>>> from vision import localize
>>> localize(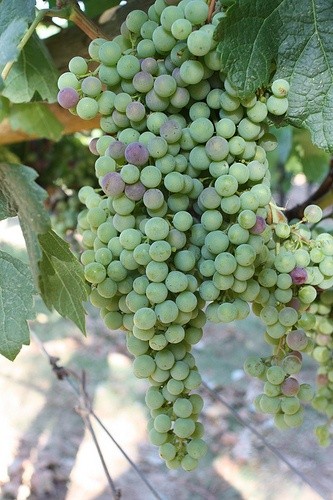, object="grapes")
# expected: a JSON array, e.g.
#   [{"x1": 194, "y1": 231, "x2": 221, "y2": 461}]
[{"x1": 11, "y1": 0, "x2": 332, "y2": 472}]
